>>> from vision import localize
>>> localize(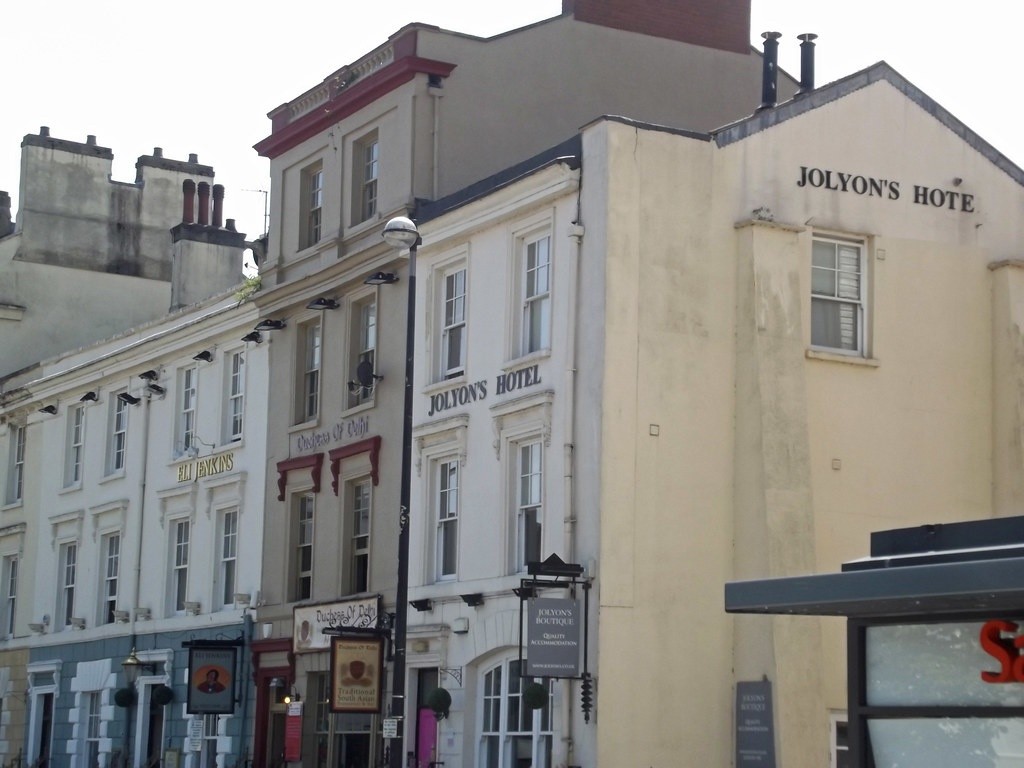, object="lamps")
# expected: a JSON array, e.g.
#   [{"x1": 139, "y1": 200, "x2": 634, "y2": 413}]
[
  {"x1": 120, "y1": 644, "x2": 141, "y2": 688},
  {"x1": 115, "y1": 369, "x2": 166, "y2": 405},
  {"x1": 193, "y1": 350, "x2": 214, "y2": 362},
  {"x1": 254, "y1": 319, "x2": 287, "y2": 332},
  {"x1": 240, "y1": 332, "x2": 263, "y2": 343},
  {"x1": 281, "y1": 685, "x2": 301, "y2": 704},
  {"x1": 409, "y1": 597, "x2": 433, "y2": 611},
  {"x1": 79, "y1": 391, "x2": 97, "y2": 402},
  {"x1": 38, "y1": 404, "x2": 58, "y2": 415},
  {"x1": 305, "y1": 296, "x2": 339, "y2": 310},
  {"x1": 365, "y1": 271, "x2": 401, "y2": 283},
  {"x1": 460, "y1": 593, "x2": 484, "y2": 607}
]
